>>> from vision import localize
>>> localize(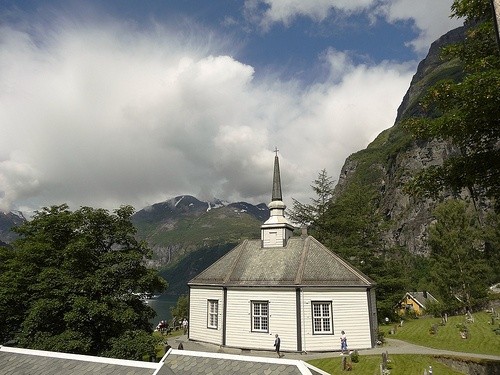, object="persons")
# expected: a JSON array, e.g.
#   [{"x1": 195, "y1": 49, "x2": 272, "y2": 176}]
[
  {"x1": 273, "y1": 334, "x2": 282, "y2": 357},
  {"x1": 182, "y1": 318, "x2": 188, "y2": 336},
  {"x1": 340, "y1": 330, "x2": 352, "y2": 356},
  {"x1": 156, "y1": 320, "x2": 169, "y2": 331}
]
